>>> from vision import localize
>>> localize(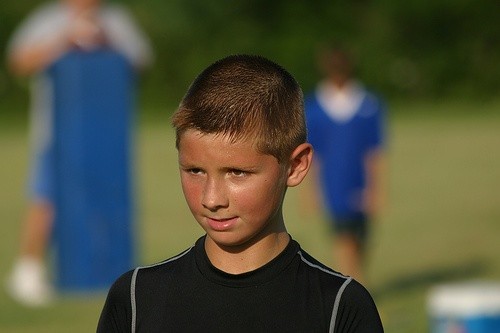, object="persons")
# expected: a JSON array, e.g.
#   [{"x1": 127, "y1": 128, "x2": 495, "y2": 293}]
[
  {"x1": 6, "y1": 0, "x2": 155, "y2": 306},
  {"x1": 303, "y1": 42, "x2": 387, "y2": 290},
  {"x1": 95, "y1": 54, "x2": 385, "y2": 333}
]
